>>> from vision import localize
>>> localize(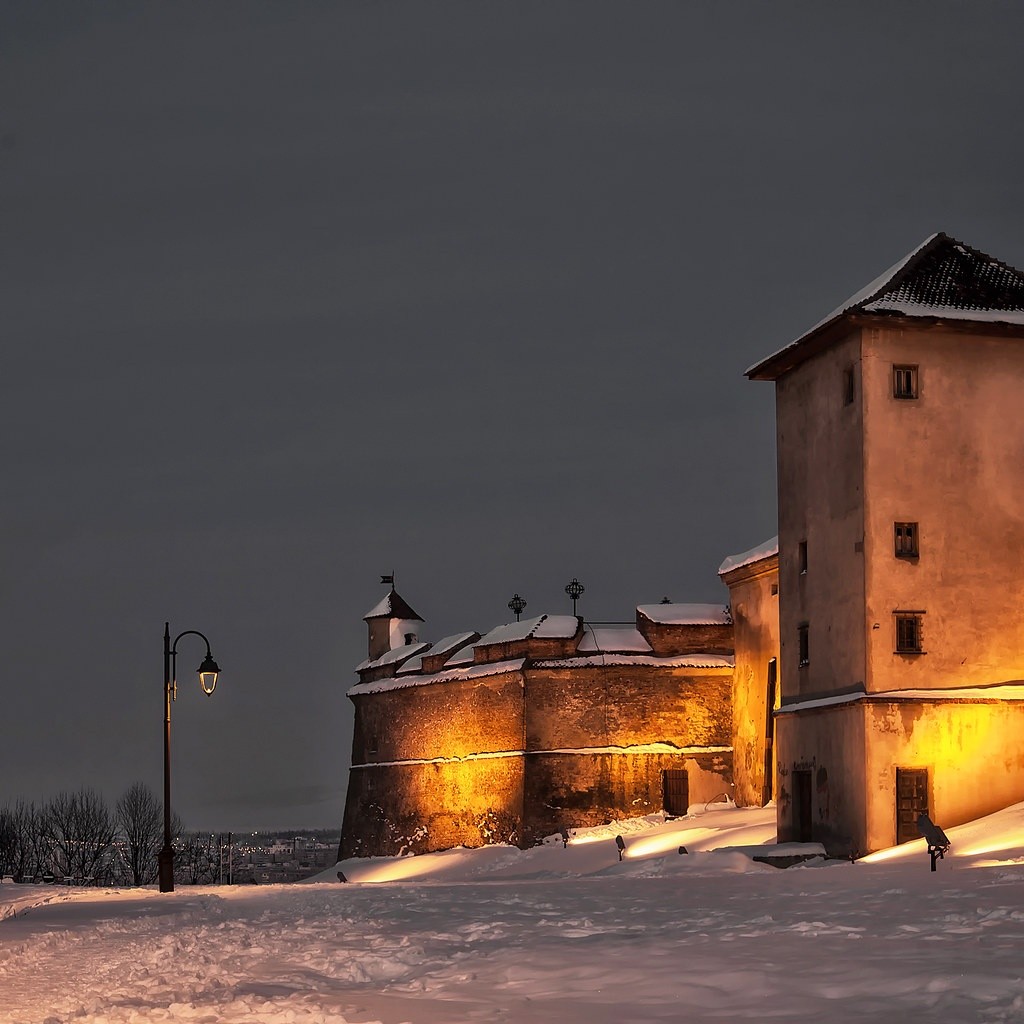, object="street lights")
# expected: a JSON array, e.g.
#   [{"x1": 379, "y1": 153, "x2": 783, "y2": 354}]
[{"x1": 159, "y1": 621, "x2": 222, "y2": 893}]
